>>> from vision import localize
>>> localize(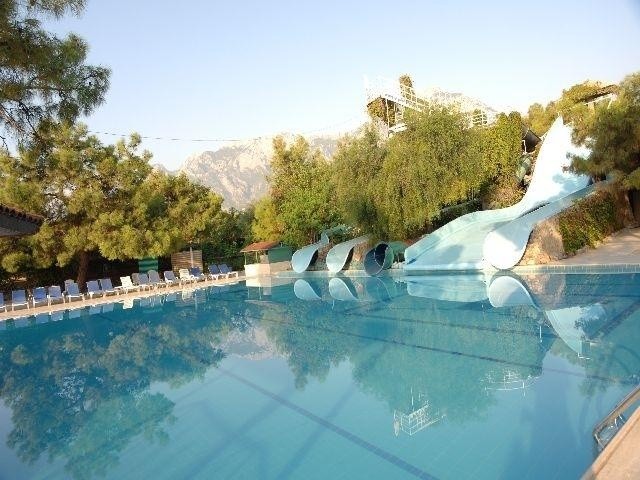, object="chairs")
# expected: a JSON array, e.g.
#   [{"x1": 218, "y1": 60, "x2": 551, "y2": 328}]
[
  {"x1": 0, "y1": 281, "x2": 238, "y2": 332},
  {"x1": 0, "y1": 264, "x2": 238, "y2": 315}
]
